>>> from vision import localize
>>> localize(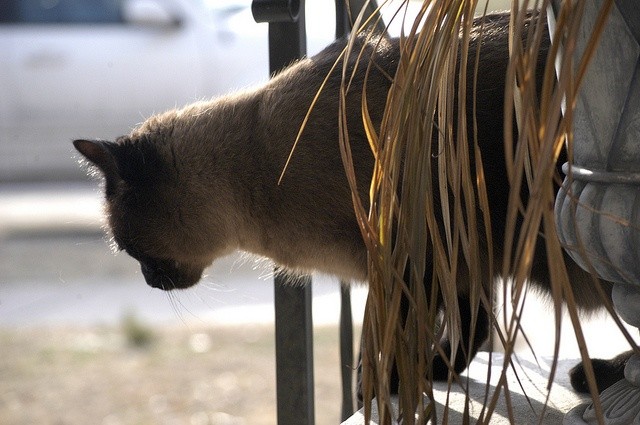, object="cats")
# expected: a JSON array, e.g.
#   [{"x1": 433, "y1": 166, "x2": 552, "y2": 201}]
[{"x1": 70, "y1": 9, "x2": 640, "y2": 400}]
